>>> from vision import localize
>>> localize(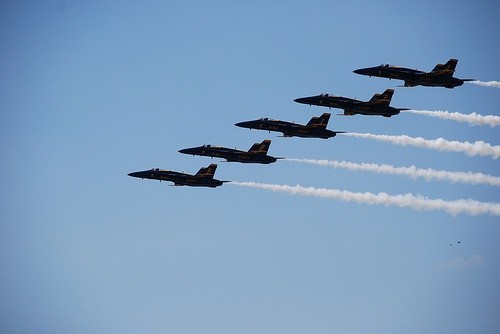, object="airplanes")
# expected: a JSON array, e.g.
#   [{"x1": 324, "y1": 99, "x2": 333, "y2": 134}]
[
  {"x1": 234, "y1": 112, "x2": 346, "y2": 139},
  {"x1": 353, "y1": 58, "x2": 476, "y2": 89},
  {"x1": 293, "y1": 89, "x2": 411, "y2": 117},
  {"x1": 126, "y1": 163, "x2": 232, "y2": 188},
  {"x1": 177, "y1": 140, "x2": 286, "y2": 164}
]
